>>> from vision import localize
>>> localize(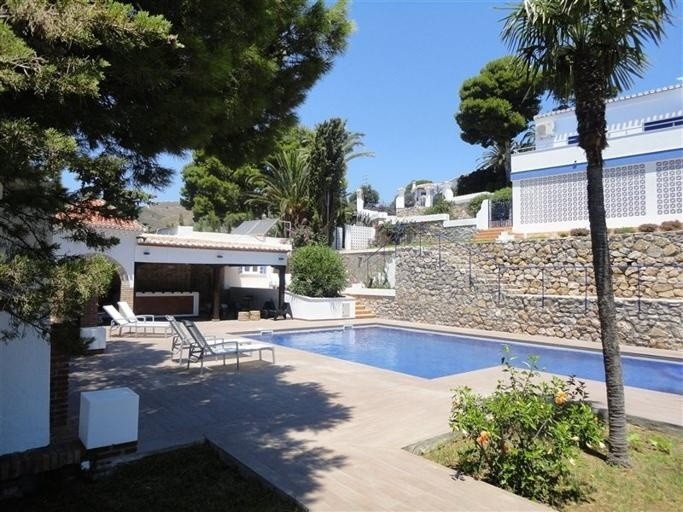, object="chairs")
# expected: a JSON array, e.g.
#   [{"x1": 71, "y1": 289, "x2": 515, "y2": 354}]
[{"x1": 102, "y1": 301, "x2": 275, "y2": 375}]
